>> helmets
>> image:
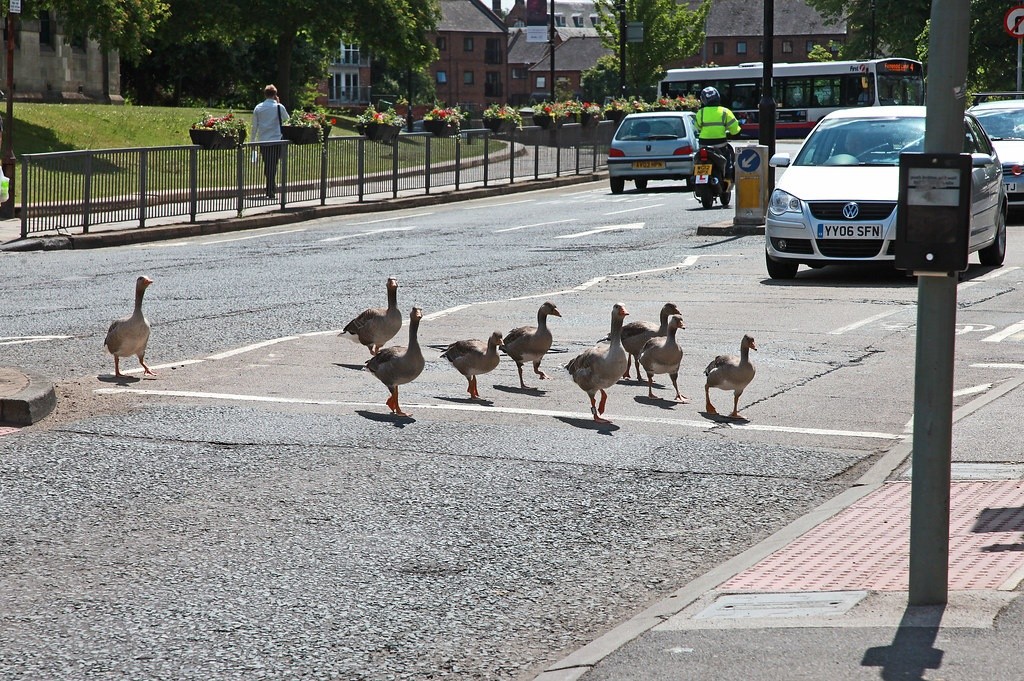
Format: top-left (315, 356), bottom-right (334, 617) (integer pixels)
top-left (700, 86), bottom-right (720, 106)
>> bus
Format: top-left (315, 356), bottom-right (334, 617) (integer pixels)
top-left (656, 57), bottom-right (926, 130)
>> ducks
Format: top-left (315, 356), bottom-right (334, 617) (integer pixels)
top-left (102, 275), bottom-right (161, 380)
top-left (637, 315), bottom-right (689, 403)
top-left (563, 302), bottom-right (630, 425)
top-left (702, 334), bottom-right (758, 420)
top-left (440, 329), bottom-right (505, 400)
top-left (498, 300), bottom-right (563, 390)
top-left (596, 302), bottom-right (683, 382)
top-left (364, 304), bottom-right (427, 418)
top-left (336, 276), bottom-right (403, 358)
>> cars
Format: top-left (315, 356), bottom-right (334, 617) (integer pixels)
top-left (965, 93), bottom-right (1024, 206)
top-left (765, 106), bottom-right (1007, 279)
top-left (607, 109), bottom-right (701, 193)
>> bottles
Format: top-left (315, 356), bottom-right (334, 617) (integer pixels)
top-left (250, 149), bottom-right (260, 162)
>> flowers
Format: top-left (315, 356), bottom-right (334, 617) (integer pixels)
top-left (483, 103), bottom-right (523, 131)
top-left (356, 104), bottom-right (406, 127)
top-left (531, 93), bottom-right (702, 122)
top-left (283, 108), bottom-right (336, 152)
top-left (423, 105), bottom-right (470, 140)
top-left (193, 106), bottom-right (246, 148)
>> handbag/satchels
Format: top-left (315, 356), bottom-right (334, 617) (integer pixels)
top-left (0, 166), bottom-right (10, 202)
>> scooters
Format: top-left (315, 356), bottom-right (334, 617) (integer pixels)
top-left (691, 119), bottom-right (747, 208)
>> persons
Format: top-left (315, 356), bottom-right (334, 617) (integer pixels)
top-left (250, 83), bottom-right (290, 201)
top-left (693, 86), bottom-right (742, 183)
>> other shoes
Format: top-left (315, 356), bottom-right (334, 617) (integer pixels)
top-left (266, 189), bottom-right (276, 198)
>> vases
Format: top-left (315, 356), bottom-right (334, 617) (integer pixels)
top-left (533, 106), bottom-right (698, 127)
top-left (354, 123), bottom-right (401, 141)
top-left (424, 121), bottom-right (464, 137)
top-left (189, 129), bottom-right (246, 148)
top-left (281, 126), bottom-right (332, 144)
top-left (482, 117), bottom-right (516, 133)
